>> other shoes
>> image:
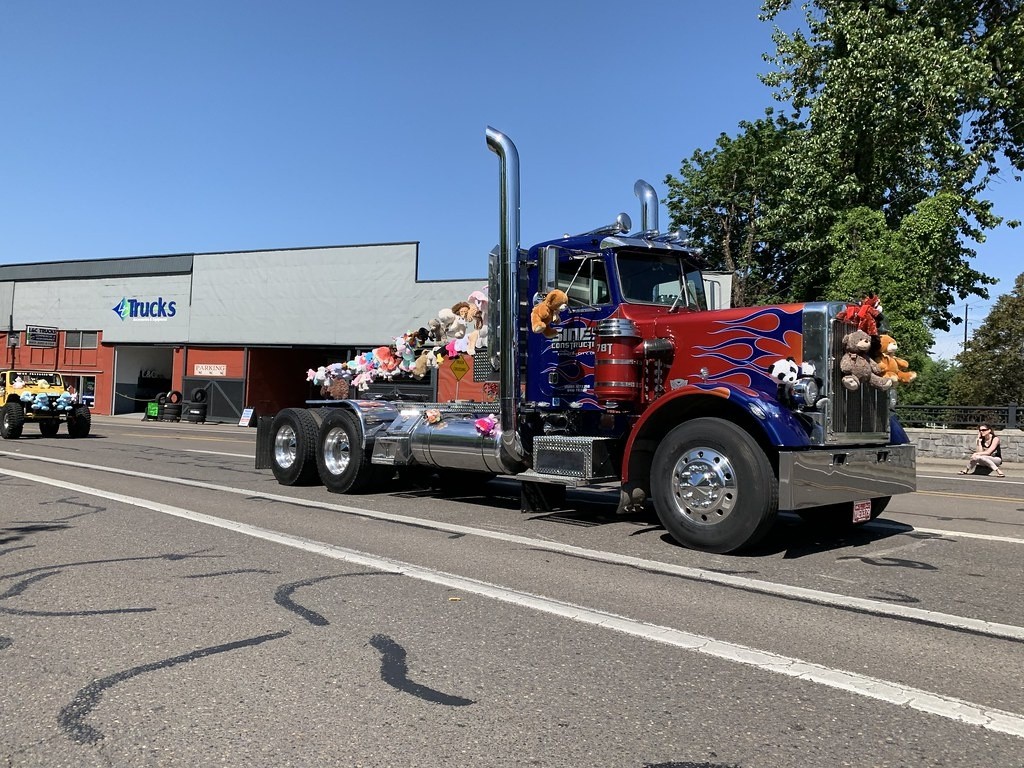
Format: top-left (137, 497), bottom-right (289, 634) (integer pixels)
top-left (957, 470), bottom-right (967, 475)
top-left (997, 473), bottom-right (1005, 477)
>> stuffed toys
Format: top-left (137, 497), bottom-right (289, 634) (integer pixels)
top-left (0, 386), bottom-right (6, 397)
top-left (306, 285), bottom-right (491, 401)
top-left (33, 379), bottom-right (50, 389)
top-left (474, 413), bottom-right (498, 436)
top-left (31, 392), bottom-right (50, 410)
top-left (858, 291), bottom-right (883, 336)
top-left (835, 304), bottom-right (859, 327)
top-left (878, 334), bottom-right (917, 387)
top-left (71, 390), bottom-right (78, 404)
top-left (56, 391), bottom-right (73, 411)
top-left (768, 356), bottom-right (823, 399)
top-left (13, 376), bottom-right (25, 389)
top-left (530, 289), bottom-right (568, 339)
top-left (425, 408), bottom-right (440, 423)
top-left (839, 329), bottom-right (893, 391)
top-left (20, 391), bottom-right (35, 401)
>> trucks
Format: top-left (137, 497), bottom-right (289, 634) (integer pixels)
top-left (255, 126), bottom-right (918, 555)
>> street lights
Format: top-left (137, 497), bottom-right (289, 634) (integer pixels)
top-left (10, 334), bottom-right (19, 369)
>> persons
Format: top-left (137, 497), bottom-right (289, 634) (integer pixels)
top-left (67, 384), bottom-right (76, 394)
top-left (957, 424), bottom-right (1006, 478)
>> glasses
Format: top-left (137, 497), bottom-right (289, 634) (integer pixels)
top-left (979, 428), bottom-right (990, 431)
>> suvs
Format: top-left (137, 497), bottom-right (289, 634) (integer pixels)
top-left (0, 369), bottom-right (91, 440)
